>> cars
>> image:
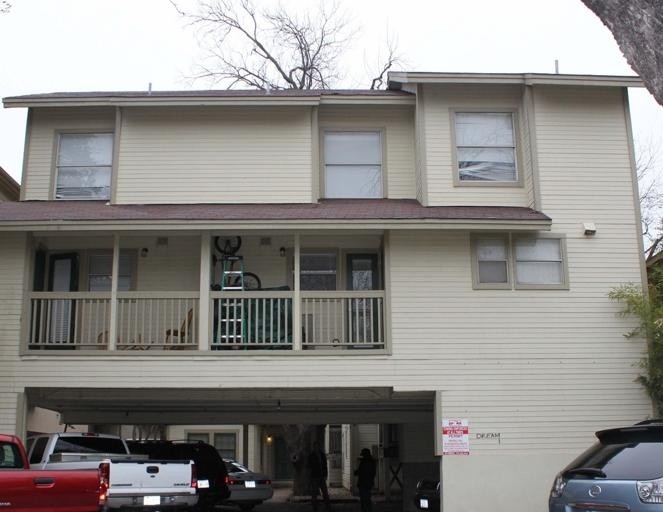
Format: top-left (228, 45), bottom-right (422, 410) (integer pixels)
top-left (219, 456), bottom-right (273, 512)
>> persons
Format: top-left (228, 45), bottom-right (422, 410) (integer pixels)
top-left (354, 448), bottom-right (376, 512)
top-left (309, 441), bottom-right (330, 512)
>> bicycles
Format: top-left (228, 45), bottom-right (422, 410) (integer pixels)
top-left (212, 235), bottom-right (261, 291)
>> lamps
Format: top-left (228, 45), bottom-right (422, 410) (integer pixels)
top-left (280, 247), bottom-right (285, 257)
top-left (584, 223), bottom-right (596, 236)
top-left (141, 248), bottom-right (148, 257)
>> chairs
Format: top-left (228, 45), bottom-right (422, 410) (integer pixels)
top-left (163, 307), bottom-right (193, 349)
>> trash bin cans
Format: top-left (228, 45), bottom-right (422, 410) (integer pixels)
top-left (414, 479), bottom-right (440, 512)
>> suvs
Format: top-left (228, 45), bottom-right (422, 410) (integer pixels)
top-left (547, 417), bottom-right (662, 512)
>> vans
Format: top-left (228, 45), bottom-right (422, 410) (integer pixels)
top-left (125, 439), bottom-right (230, 512)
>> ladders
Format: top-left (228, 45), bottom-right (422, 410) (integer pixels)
top-left (215, 256), bottom-right (247, 350)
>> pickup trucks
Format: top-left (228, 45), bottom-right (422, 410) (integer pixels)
top-left (25, 433), bottom-right (199, 512)
top-left (0, 432), bottom-right (109, 512)
